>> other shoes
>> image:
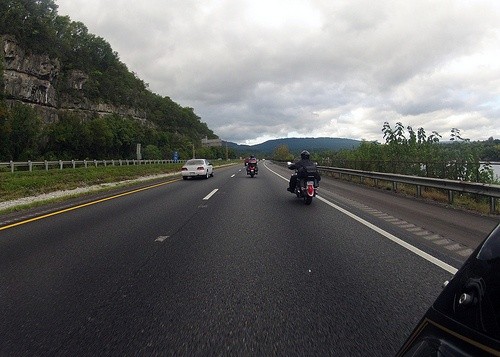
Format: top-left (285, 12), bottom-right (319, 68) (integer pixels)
top-left (287, 188), bottom-right (294, 192)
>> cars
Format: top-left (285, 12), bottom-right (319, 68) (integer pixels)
top-left (244, 157), bottom-right (250, 166)
top-left (181, 159), bottom-right (214, 180)
top-left (262, 159), bottom-right (265, 161)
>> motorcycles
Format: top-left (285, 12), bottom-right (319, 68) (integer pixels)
top-left (287, 162), bottom-right (320, 205)
top-left (246, 159), bottom-right (258, 178)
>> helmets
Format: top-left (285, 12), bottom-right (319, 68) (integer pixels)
top-left (301, 150), bottom-right (310, 155)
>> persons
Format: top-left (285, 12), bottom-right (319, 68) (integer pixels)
top-left (286, 149), bottom-right (318, 195)
top-left (246, 154), bottom-right (258, 175)
